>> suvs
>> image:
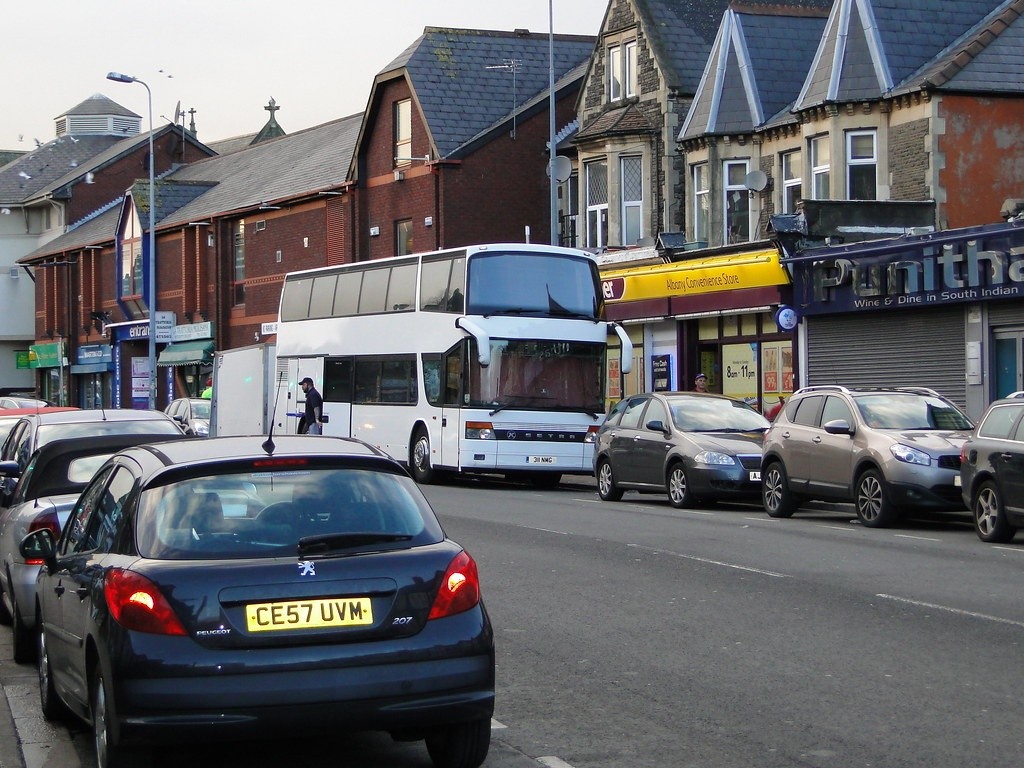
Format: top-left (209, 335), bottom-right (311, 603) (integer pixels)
top-left (760, 385), bottom-right (976, 527)
top-left (960, 384), bottom-right (1024, 545)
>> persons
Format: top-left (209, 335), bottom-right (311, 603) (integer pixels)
top-left (690, 373), bottom-right (710, 394)
top-left (297, 376), bottom-right (324, 435)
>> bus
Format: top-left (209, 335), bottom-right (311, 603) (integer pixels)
top-left (269, 243), bottom-right (632, 486)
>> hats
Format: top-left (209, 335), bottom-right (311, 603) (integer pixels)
top-left (696, 373), bottom-right (708, 379)
top-left (298, 378), bottom-right (312, 385)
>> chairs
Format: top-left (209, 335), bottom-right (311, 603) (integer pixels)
top-left (291, 480), bottom-right (354, 532)
top-left (173, 491), bottom-right (225, 535)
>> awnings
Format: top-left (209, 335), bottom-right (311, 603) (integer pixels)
top-left (157, 341), bottom-right (215, 368)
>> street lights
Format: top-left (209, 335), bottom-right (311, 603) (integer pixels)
top-left (107, 71), bottom-right (156, 411)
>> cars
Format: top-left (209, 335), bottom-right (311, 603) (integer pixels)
top-left (164, 398), bottom-right (211, 437)
top-left (0, 398), bottom-right (188, 669)
top-left (19, 435), bottom-right (496, 768)
top-left (593, 391), bottom-right (771, 509)
